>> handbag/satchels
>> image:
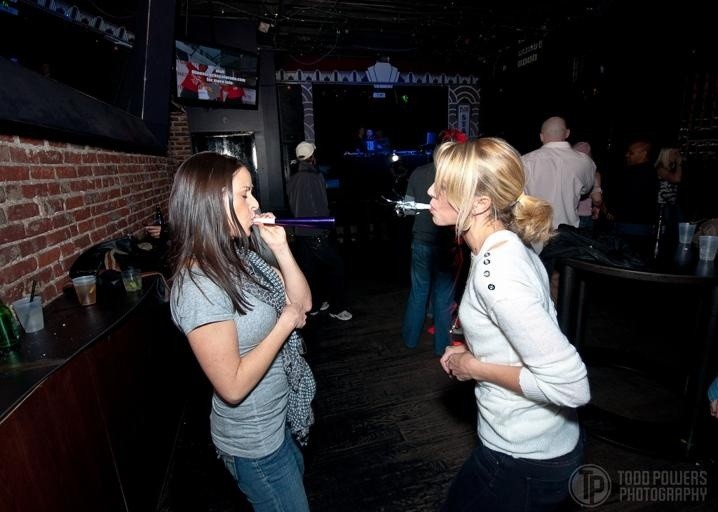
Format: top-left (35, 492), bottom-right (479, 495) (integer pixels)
top-left (539, 221), bottom-right (655, 270)
top-left (68, 235), bottom-right (173, 293)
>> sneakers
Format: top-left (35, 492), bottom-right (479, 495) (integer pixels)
top-left (329, 310), bottom-right (354, 321)
top-left (309, 300), bottom-right (330, 317)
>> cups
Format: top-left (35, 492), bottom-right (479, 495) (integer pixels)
top-left (679, 222), bottom-right (696, 243)
top-left (676, 242), bottom-right (692, 263)
top-left (12, 295), bottom-right (44, 334)
top-left (121, 266), bottom-right (142, 292)
top-left (699, 235), bottom-right (718, 261)
top-left (696, 260), bottom-right (716, 277)
top-left (71, 275), bottom-right (97, 306)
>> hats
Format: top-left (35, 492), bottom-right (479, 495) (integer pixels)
top-left (295, 141), bottom-right (318, 162)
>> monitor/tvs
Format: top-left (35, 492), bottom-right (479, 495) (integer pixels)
top-left (173, 37), bottom-right (260, 110)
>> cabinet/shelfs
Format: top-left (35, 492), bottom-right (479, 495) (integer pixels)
top-left (0, 279), bottom-right (221, 512)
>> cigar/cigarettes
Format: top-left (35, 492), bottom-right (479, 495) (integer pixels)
top-left (414, 203), bottom-right (431, 210)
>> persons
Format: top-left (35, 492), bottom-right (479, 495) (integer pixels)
top-left (400, 116), bottom-right (718, 356)
top-left (426, 137), bottom-right (592, 512)
top-left (144, 160), bottom-right (182, 245)
top-left (166, 151), bottom-right (315, 512)
top-left (286, 141), bottom-right (354, 321)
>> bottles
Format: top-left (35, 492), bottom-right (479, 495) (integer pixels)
top-left (653, 204), bottom-right (666, 242)
top-left (155, 203), bottom-right (165, 225)
top-left (0, 298), bottom-right (21, 349)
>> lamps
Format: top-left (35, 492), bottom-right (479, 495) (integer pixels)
top-left (366, 57), bottom-right (401, 88)
